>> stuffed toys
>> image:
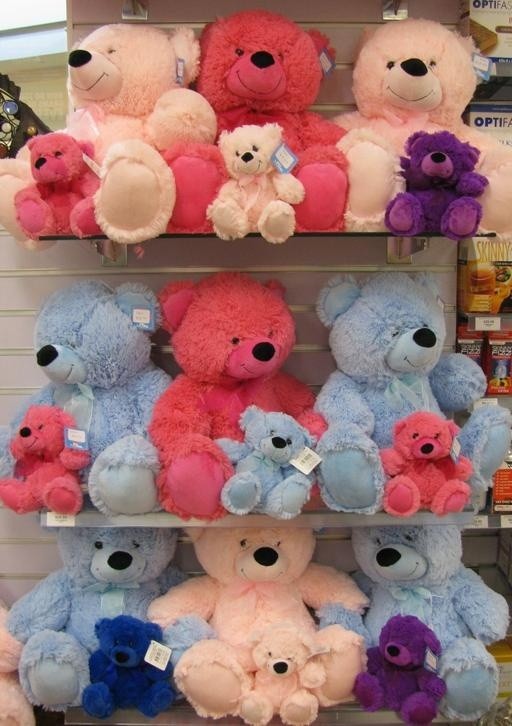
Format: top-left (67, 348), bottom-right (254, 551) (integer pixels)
top-left (212, 405), bottom-right (319, 522)
top-left (16, 132), bottom-right (103, 239)
top-left (149, 272), bottom-right (327, 522)
top-left (6, 528), bottom-right (216, 713)
top-left (239, 622), bottom-right (326, 726)
top-left (206, 122), bottom-right (305, 244)
top-left (385, 128), bottom-right (488, 240)
top-left (1, 404), bottom-right (90, 517)
top-left (161, 9), bottom-right (349, 236)
top-left (353, 614), bottom-right (446, 725)
top-left (1, 603), bottom-right (37, 726)
top-left (314, 268), bottom-right (511, 516)
top-left (378, 412), bottom-right (472, 516)
top-left (82, 615), bottom-right (176, 718)
top-left (318, 525), bottom-right (509, 722)
top-left (328, 16), bottom-right (511, 238)
top-left (1, 21), bottom-right (217, 252)
top-left (148, 526), bottom-right (370, 721)
top-left (1, 278), bottom-right (173, 518)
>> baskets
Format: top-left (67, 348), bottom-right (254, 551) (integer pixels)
top-left (494, 468), bottom-right (512, 502)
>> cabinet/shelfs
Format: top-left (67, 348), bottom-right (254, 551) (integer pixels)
top-left (3, 213), bottom-right (512, 726)
